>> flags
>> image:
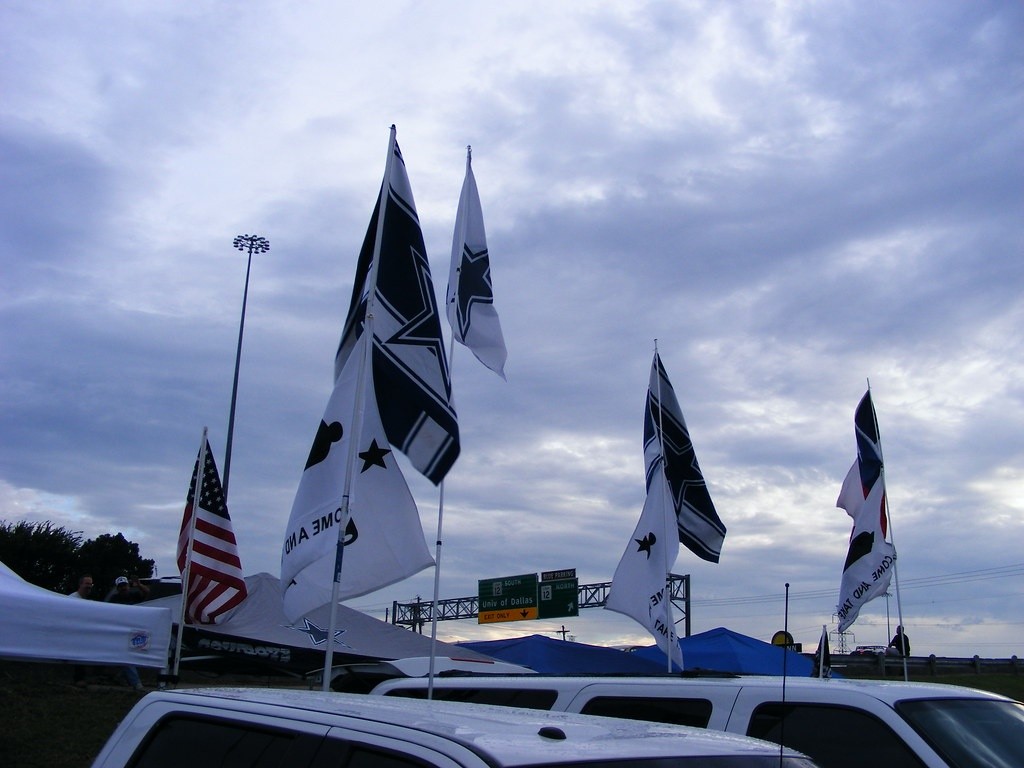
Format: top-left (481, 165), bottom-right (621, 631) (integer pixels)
top-left (444, 164), bottom-right (510, 381)
top-left (176, 437), bottom-right (248, 624)
top-left (280, 140), bottom-right (461, 624)
top-left (836, 389), bottom-right (894, 633)
top-left (603, 349), bottom-right (727, 670)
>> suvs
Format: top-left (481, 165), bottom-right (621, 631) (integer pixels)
top-left (323, 655), bottom-right (530, 708)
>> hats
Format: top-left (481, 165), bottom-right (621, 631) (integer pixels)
top-left (115, 576), bottom-right (128, 590)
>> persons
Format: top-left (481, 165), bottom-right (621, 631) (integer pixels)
top-left (68, 574), bottom-right (152, 700)
top-left (886, 624), bottom-right (911, 658)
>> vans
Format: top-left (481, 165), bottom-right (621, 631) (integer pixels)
top-left (88, 686), bottom-right (812, 768)
top-left (372, 672), bottom-right (1024, 768)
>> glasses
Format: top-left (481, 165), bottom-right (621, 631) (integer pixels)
top-left (82, 583), bottom-right (94, 586)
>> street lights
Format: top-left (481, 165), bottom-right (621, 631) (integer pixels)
top-left (222, 233), bottom-right (271, 502)
